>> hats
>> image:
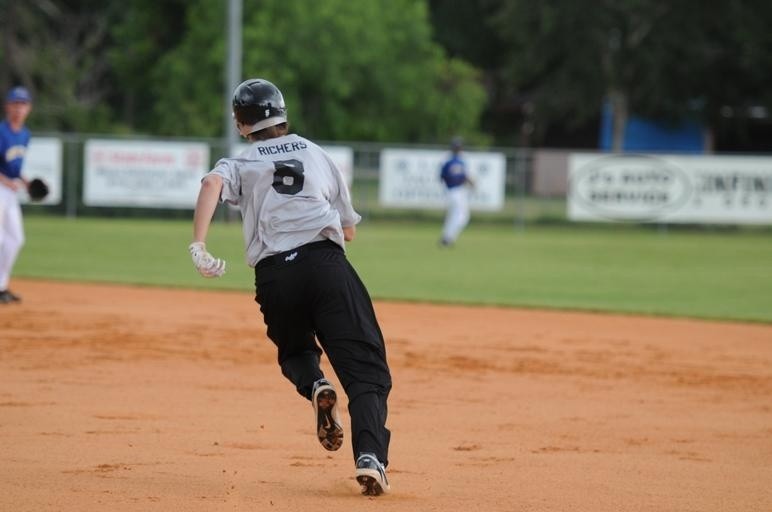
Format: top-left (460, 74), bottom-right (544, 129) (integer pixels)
top-left (8, 87), bottom-right (29, 103)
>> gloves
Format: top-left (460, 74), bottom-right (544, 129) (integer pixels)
top-left (188, 242), bottom-right (226, 277)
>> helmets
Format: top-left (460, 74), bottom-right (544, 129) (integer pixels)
top-left (232, 79), bottom-right (287, 136)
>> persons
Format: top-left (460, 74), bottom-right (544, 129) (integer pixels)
top-left (189, 78), bottom-right (393, 496)
top-left (0, 86), bottom-right (35, 302)
top-left (439, 138), bottom-right (476, 245)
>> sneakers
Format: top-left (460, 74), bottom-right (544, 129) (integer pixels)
top-left (354, 454), bottom-right (389, 495)
top-left (311, 378), bottom-right (344, 451)
top-left (0, 292), bottom-right (21, 301)
